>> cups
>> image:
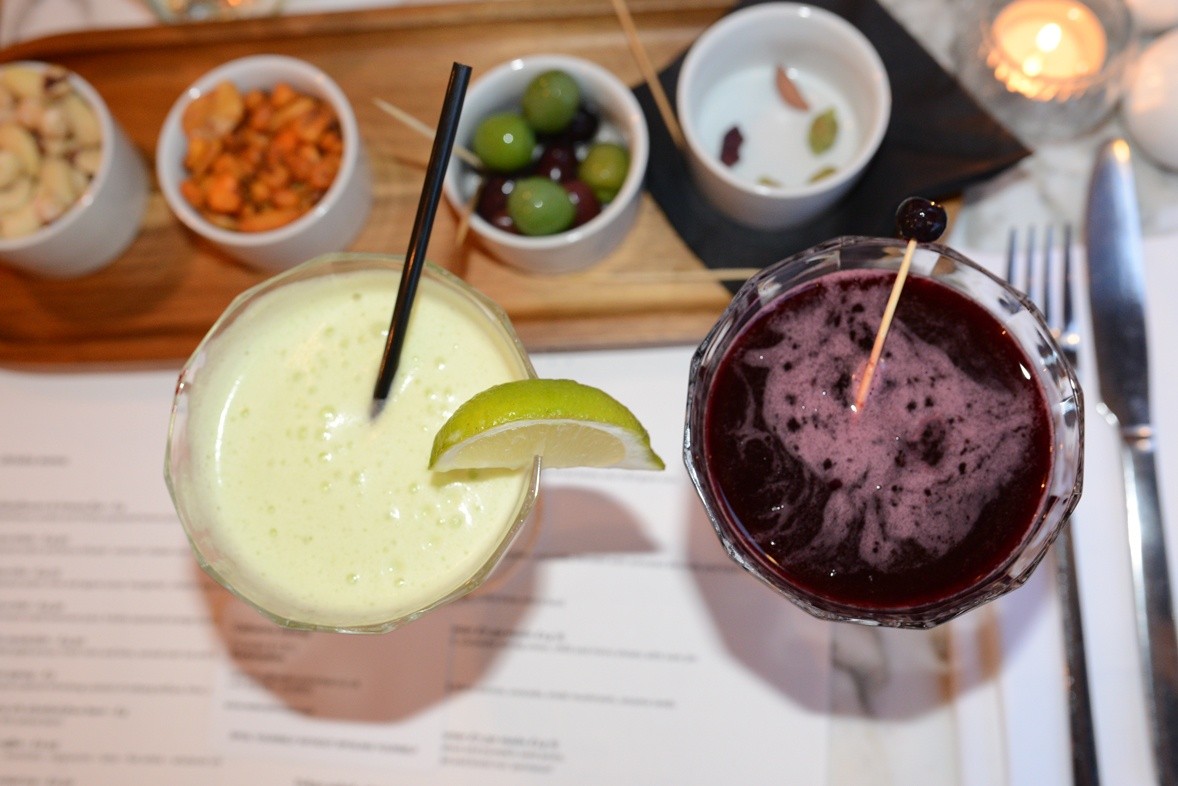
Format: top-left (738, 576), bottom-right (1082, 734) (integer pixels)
top-left (163, 251), bottom-right (538, 636)
top-left (684, 233), bottom-right (1084, 631)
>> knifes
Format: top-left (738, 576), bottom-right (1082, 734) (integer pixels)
top-left (1086, 135), bottom-right (1178, 786)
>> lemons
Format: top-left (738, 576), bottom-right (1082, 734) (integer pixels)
top-left (423, 378), bottom-right (667, 471)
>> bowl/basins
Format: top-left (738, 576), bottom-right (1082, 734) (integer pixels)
top-left (676, 0), bottom-right (891, 234)
top-left (1, 60), bottom-right (146, 276)
top-left (442, 57), bottom-right (650, 276)
top-left (155, 54), bottom-right (369, 273)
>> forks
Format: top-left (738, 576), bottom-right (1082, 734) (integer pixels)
top-left (1004, 222), bottom-right (1102, 785)
